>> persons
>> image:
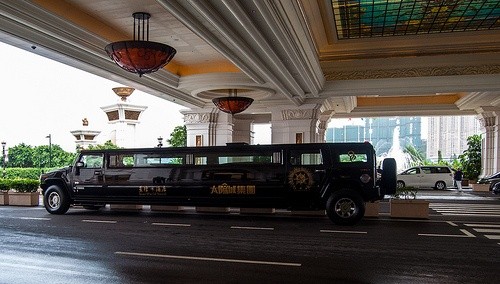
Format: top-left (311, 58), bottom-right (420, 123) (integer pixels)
top-left (454, 168), bottom-right (464, 192)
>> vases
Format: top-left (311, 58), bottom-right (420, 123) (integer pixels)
top-left (378, 197), bottom-right (390, 213)
top-left (364, 201), bottom-right (378, 216)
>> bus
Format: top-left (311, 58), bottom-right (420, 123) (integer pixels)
top-left (38, 140), bottom-right (397, 228)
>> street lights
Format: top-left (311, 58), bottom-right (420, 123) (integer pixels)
top-left (45, 133), bottom-right (51, 167)
top-left (0, 141), bottom-right (7, 179)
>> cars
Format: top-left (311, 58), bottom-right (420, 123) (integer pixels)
top-left (475, 171), bottom-right (500, 196)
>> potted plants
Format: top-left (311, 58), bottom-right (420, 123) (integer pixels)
top-left (0, 176), bottom-right (10, 206)
top-left (471, 178), bottom-right (492, 191)
top-left (7, 176), bottom-right (40, 206)
top-left (390, 184), bottom-right (429, 219)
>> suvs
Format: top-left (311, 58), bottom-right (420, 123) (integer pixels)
top-left (395, 165), bottom-right (455, 191)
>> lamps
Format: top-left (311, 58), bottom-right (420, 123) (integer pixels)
top-left (210, 88), bottom-right (254, 115)
top-left (104, 12), bottom-right (176, 78)
